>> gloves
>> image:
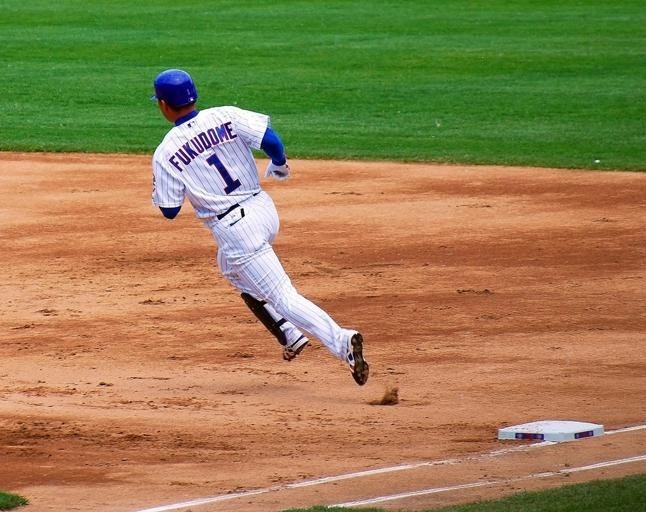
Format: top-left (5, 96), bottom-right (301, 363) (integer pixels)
top-left (264, 162), bottom-right (290, 180)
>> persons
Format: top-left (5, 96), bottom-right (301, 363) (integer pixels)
top-left (149, 69), bottom-right (370, 386)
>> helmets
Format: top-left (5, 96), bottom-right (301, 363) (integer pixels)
top-left (151, 69), bottom-right (197, 106)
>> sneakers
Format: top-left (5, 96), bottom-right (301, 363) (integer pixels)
top-left (345, 331), bottom-right (369, 385)
top-left (283, 335), bottom-right (308, 360)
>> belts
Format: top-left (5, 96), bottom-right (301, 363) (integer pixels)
top-left (217, 203), bottom-right (240, 219)
